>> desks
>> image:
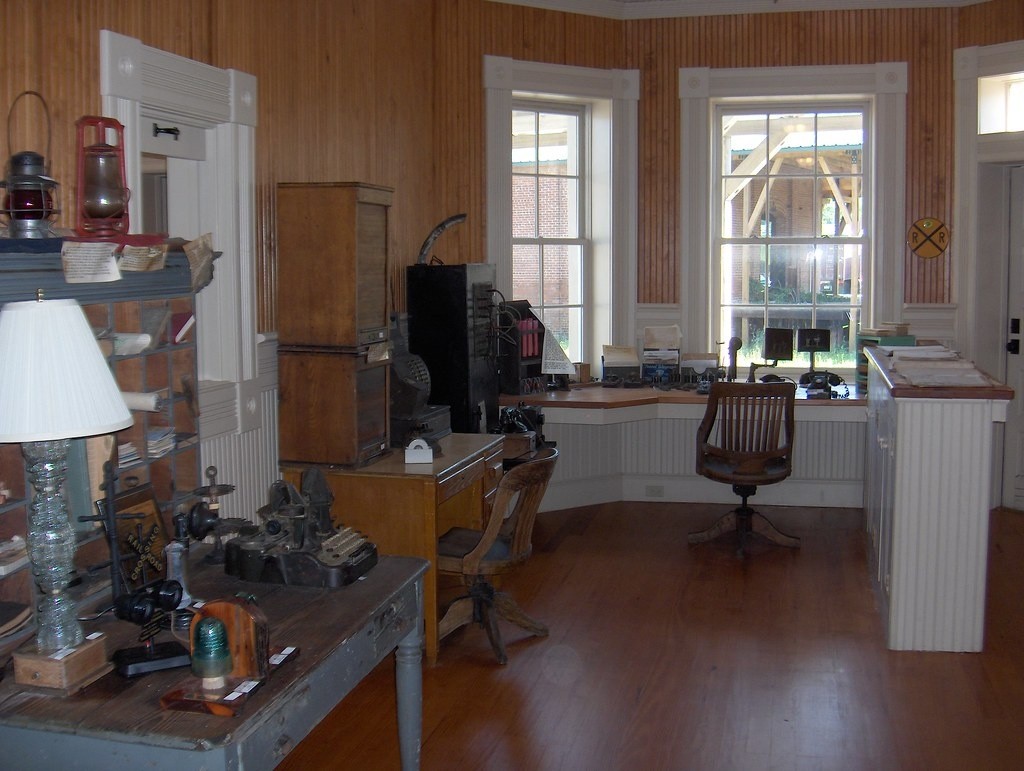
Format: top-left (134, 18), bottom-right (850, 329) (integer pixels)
top-left (500, 383), bottom-right (870, 517)
top-left (282, 432), bottom-right (507, 665)
top-left (1, 548), bottom-right (430, 771)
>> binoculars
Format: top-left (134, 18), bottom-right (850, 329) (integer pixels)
top-left (111, 578), bottom-right (184, 627)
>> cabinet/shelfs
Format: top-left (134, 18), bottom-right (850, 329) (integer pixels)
top-left (862, 346), bottom-right (1014, 653)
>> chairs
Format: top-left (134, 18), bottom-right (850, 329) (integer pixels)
top-left (393, 447), bottom-right (560, 666)
top-left (686, 382), bottom-right (803, 551)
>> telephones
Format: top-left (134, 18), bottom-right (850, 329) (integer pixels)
top-left (799, 370), bottom-right (841, 400)
top-left (498, 406), bottom-right (528, 434)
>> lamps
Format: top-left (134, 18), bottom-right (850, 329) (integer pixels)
top-left (0, 288), bottom-right (134, 698)
top-left (2, 92), bottom-right (65, 243)
top-left (74, 116), bottom-right (131, 241)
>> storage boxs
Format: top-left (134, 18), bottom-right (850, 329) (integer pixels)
top-left (275, 179), bottom-right (395, 469)
top-left (391, 406), bottom-right (454, 449)
top-left (505, 431), bottom-right (538, 461)
